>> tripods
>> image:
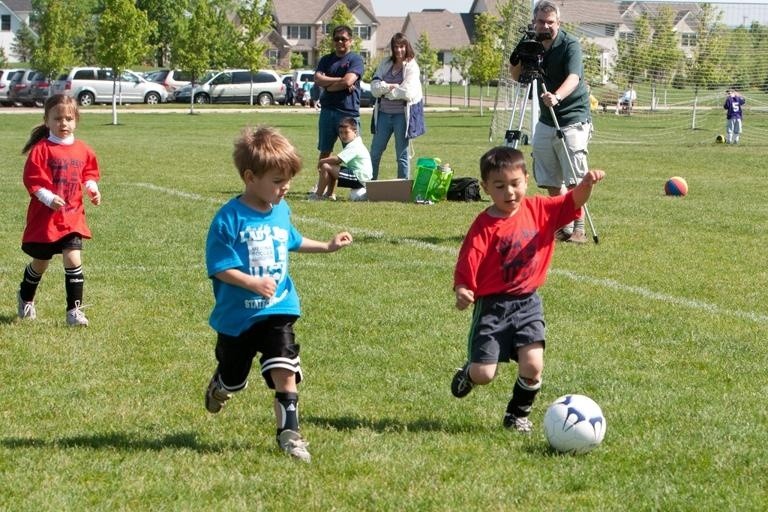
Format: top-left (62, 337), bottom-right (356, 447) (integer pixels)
top-left (504, 71), bottom-right (600, 244)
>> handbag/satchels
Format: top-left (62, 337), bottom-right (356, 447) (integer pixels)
top-left (408, 162), bottom-right (454, 205)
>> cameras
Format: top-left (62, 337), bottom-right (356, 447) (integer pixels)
top-left (516, 24), bottom-right (552, 70)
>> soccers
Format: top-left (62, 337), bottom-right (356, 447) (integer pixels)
top-left (664, 176), bottom-right (689, 198)
top-left (715, 135), bottom-right (727, 145)
top-left (348, 188), bottom-right (367, 202)
top-left (542, 392), bottom-right (607, 456)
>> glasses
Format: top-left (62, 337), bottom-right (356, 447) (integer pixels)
top-left (332, 36), bottom-right (350, 42)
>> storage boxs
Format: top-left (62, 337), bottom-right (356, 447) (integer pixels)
top-left (366, 180), bottom-right (415, 202)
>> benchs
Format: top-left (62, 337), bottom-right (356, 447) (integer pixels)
top-left (598, 92), bottom-right (634, 115)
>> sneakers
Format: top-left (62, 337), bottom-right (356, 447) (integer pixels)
top-left (329, 193), bottom-right (337, 200)
top-left (307, 183), bottom-right (328, 195)
top-left (304, 193), bottom-right (325, 200)
top-left (205, 371), bottom-right (232, 413)
top-left (16, 289), bottom-right (37, 321)
top-left (65, 299), bottom-right (94, 326)
top-left (279, 429), bottom-right (312, 464)
top-left (553, 230), bottom-right (571, 241)
top-left (451, 360), bottom-right (475, 398)
top-left (566, 230), bottom-right (589, 243)
top-left (503, 412), bottom-right (537, 439)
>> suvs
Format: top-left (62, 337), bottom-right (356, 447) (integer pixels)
top-left (9, 70), bottom-right (37, 106)
top-left (294, 70), bottom-right (376, 107)
top-left (143, 70), bottom-right (170, 85)
top-left (0, 68), bottom-right (24, 106)
top-left (173, 68), bottom-right (286, 106)
top-left (52, 67), bottom-right (169, 106)
top-left (31, 71), bottom-right (58, 108)
top-left (163, 69), bottom-right (218, 100)
top-left (282, 75), bottom-right (293, 85)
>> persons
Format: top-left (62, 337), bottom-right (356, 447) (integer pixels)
top-left (586, 85), bottom-right (599, 111)
top-left (302, 77), bottom-right (312, 108)
top-left (305, 117), bottom-right (373, 201)
top-left (510, 1), bottom-right (592, 245)
top-left (308, 25), bottom-right (364, 194)
top-left (724, 89), bottom-right (745, 143)
top-left (370, 33), bottom-right (426, 179)
top-left (203, 128), bottom-right (353, 466)
top-left (311, 84), bottom-right (321, 109)
top-left (16, 94), bottom-right (102, 328)
top-left (451, 148), bottom-right (605, 432)
top-left (616, 80), bottom-right (637, 117)
top-left (284, 78), bottom-right (295, 106)
